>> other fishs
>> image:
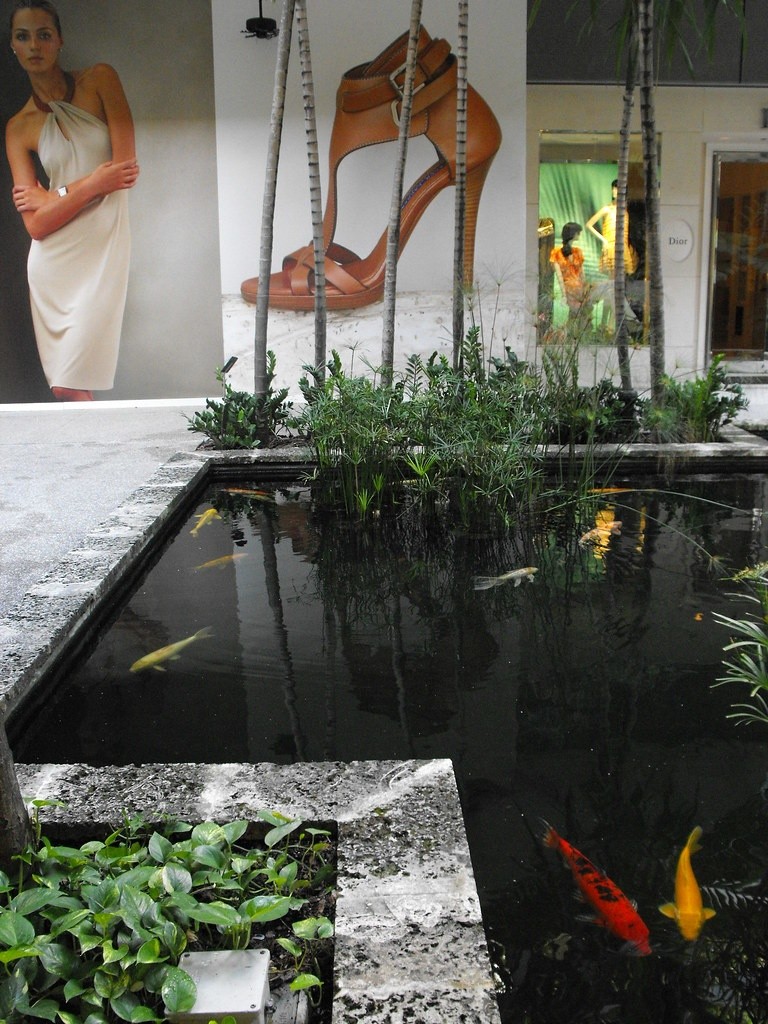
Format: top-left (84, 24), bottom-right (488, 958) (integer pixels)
top-left (129, 625), bottom-right (216, 673)
top-left (578, 521), bottom-right (623, 551)
top-left (534, 814), bottom-right (652, 958)
top-left (187, 552), bottom-right (248, 578)
top-left (189, 508), bottom-right (222, 537)
top-left (657, 825), bottom-right (717, 942)
top-left (587, 486), bottom-right (656, 496)
top-left (218, 487), bottom-right (275, 502)
top-left (470, 566), bottom-right (539, 590)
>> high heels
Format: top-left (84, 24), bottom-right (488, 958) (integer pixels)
top-left (240, 24), bottom-right (503, 312)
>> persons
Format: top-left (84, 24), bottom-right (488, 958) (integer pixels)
top-left (550, 222), bottom-right (594, 330)
top-left (586, 179), bottom-right (633, 284)
top-left (3, 0), bottom-right (139, 404)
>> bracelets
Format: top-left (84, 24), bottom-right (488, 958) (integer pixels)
top-left (57, 186), bottom-right (67, 197)
